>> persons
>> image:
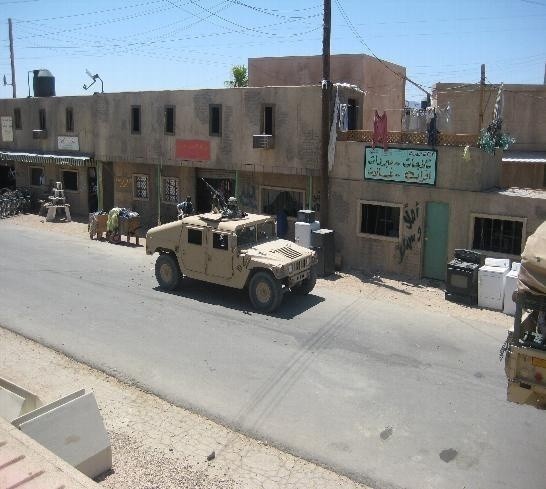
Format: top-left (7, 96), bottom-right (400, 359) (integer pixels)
top-left (218, 196), bottom-right (241, 219)
top-left (176, 196), bottom-right (194, 218)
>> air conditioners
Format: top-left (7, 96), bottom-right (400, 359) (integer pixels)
top-left (254, 135), bottom-right (273, 149)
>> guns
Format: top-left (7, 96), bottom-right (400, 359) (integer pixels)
top-left (201, 177), bottom-right (227, 214)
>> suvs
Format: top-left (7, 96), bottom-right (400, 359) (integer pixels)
top-left (145, 177), bottom-right (318, 316)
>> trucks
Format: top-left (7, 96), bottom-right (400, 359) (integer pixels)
top-left (497, 219), bottom-right (544, 410)
